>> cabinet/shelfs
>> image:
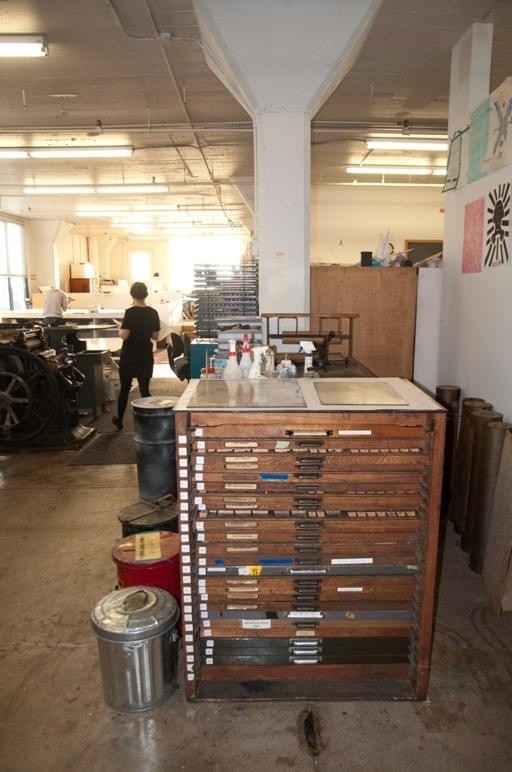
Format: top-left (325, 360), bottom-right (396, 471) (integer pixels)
top-left (172, 378), bottom-right (448, 701)
top-left (2, 309), bottom-right (125, 338)
top-left (261, 313), bottom-right (359, 366)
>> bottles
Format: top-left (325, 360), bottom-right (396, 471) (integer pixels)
top-left (223, 351), bottom-right (243, 380)
top-left (239, 349), bottom-right (253, 377)
top-left (276, 361), bottom-right (296, 378)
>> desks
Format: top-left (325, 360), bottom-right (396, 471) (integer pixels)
top-left (79, 338), bottom-right (125, 401)
top-left (190, 337), bottom-right (218, 379)
top-left (297, 357), bottom-right (377, 377)
top-left (65, 324), bottom-right (116, 338)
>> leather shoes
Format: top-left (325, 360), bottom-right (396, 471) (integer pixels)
top-left (113, 416), bottom-right (122, 429)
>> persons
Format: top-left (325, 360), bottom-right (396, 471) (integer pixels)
top-left (42, 289), bottom-right (68, 326)
top-left (151, 272), bottom-right (161, 292)
top-left (112, 282), bottom-right (160, 429)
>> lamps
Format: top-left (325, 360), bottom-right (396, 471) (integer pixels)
top-left (20, 168), bottom-right (169, 195)
top-left (363, 134), bottom-right (448, 151)
top-left (343, 149), bottom-right (447, 176)
top-left (0, 35), bottom-right (49, 57)
top-left (0, 145), bottom-right (134, 159)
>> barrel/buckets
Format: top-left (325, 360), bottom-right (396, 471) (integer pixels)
top-left (127, 395), bottom-right (176, 502)
top-left (111, 494), bottom-right (180, 594)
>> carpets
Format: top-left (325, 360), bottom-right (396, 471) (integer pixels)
top-left (84, 378), bottom-right (188, 432)
top-left (64, 433), bottom-right (137, 465)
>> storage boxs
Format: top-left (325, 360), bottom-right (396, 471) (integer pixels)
top-left (71, 278), bottom-right (95, 293)
top-left (70, 263), bottom-right (94, 278)
